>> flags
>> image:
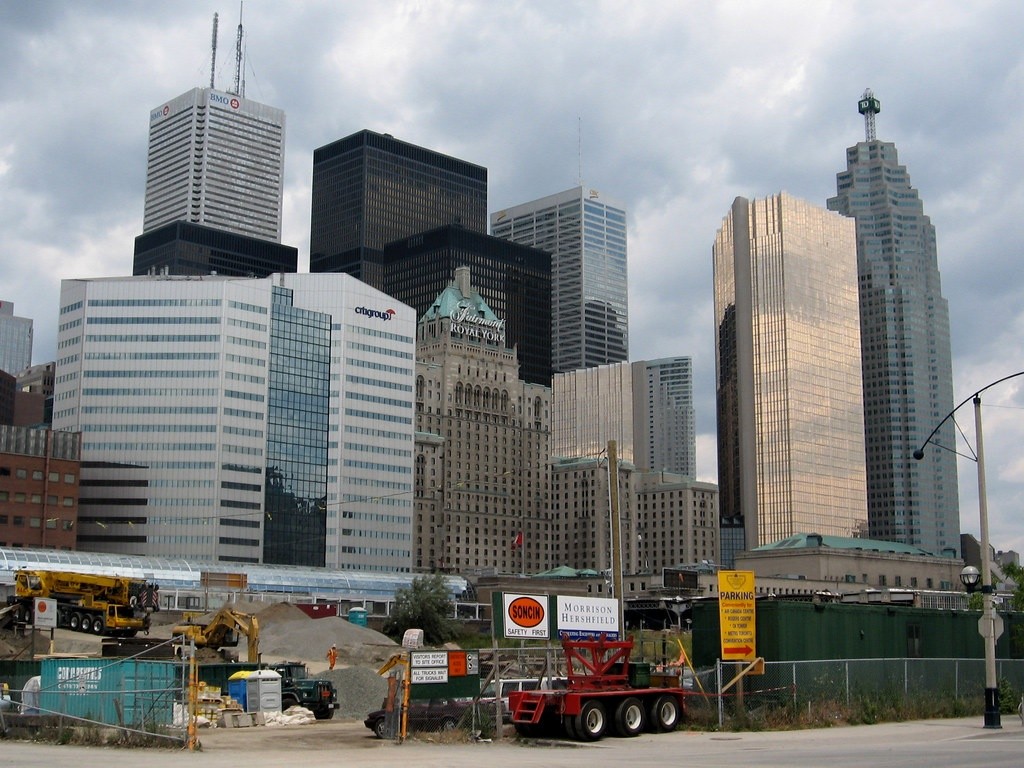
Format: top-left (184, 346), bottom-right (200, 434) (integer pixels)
top-left (511, 530), bottom-right (522, 546)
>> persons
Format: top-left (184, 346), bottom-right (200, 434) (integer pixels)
top-left (325, 643), bottom-right (339, 671)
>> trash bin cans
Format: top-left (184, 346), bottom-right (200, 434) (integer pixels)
top-left (228, 670), bottom-right (258, 712)
top-left (245, 670), bottom-right (282, 714)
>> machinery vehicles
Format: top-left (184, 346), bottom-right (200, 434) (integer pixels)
top-left (507, 633), bottom-right (684, 743)
top-left (171, 607), bottom-right (340, 720)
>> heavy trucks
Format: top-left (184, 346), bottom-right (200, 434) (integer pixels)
top-left (6, 569), bottom-right (159, 637)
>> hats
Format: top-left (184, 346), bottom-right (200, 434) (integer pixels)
top-left (332, 644), bottom-right (336, 647)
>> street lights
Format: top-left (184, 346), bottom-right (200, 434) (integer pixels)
top-left (959, 566), bottom-right (1003, 729)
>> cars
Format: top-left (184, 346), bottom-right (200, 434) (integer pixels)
top-left (364, 697), bottom-right (506, 739)
top-left (444, 678), bottom-right (568, 711)
